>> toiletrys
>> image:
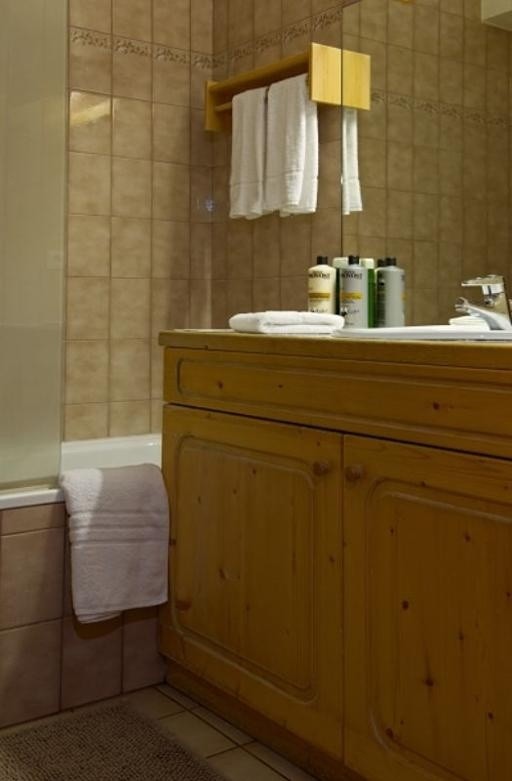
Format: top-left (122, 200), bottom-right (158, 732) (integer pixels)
top-left (306, 255), bottom-right (406, 328)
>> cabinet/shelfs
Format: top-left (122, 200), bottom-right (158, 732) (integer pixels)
top-left (160, 330), bottom-right (511, 781)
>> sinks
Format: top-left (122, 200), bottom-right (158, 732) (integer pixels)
top-left (332, 322), bottom-right (512, 341)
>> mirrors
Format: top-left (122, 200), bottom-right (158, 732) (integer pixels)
top-left (341, 0), bottom-right (512, 329)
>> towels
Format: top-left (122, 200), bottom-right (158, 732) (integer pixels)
top-left (228, 86), bottom-right (274, 220)
top-left (57, 464), bottom-right (169, 624)
top-left (262, 71), bottom-right (319, 218)
top-left (228, 311), bottom-right (344, 335)
top-left (449, 315), bottom-right (489, 329)
top-left (341, 107), bottom-right (363, 216)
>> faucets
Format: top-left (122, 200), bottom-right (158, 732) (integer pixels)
top-left (454, 274), bottom-right (511, 331)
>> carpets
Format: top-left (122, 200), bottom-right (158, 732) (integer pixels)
top-left (0, 700), bottom-right (228, 781)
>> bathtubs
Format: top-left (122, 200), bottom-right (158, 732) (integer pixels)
top-left (0, 432), bottom-right (162, 509)
